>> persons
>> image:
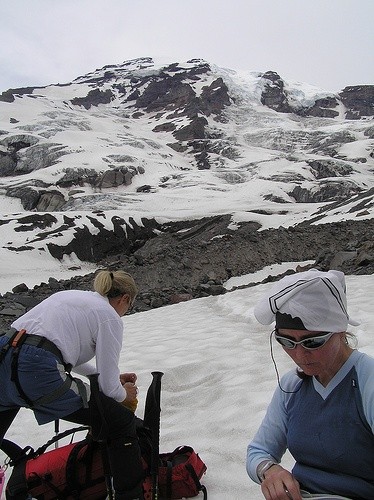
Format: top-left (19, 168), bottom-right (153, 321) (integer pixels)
top-left (0, 271), bottom-right (142, 499)
top-left (245, 268), bottom-right (374, 499)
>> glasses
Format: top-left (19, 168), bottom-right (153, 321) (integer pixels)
top-left (274, 331), bottom-right (334, 350)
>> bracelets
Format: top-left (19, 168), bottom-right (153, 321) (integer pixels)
top-left (257, 461), bottom-right (278, 479)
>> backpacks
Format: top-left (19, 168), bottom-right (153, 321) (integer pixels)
top-left (0, 416), bottom-right (208, 500)
